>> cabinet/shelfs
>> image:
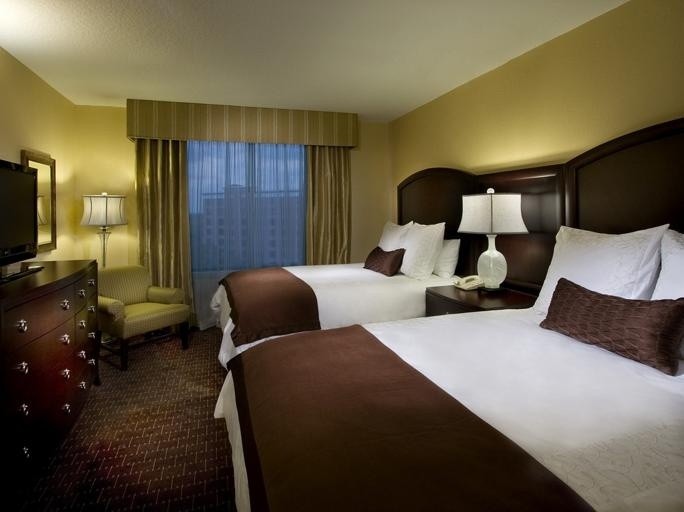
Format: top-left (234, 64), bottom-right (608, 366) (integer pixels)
top-left (0, 259), bottom-right (100, 511)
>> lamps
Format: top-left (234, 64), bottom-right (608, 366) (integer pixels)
top-left (77, 192), bottom-right (129, 267)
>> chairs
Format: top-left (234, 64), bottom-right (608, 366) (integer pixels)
top-left (98, 264), bottom-right (193, 371)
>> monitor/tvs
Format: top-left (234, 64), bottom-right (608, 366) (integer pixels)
top-left (0, 159), bottom-right (44, 285)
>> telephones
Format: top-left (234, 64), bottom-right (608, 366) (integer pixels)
top-left (453, 275), bottom-right (481, 291)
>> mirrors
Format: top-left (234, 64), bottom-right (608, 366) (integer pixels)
top-left (18, 145), bottom-right (57, 255)
